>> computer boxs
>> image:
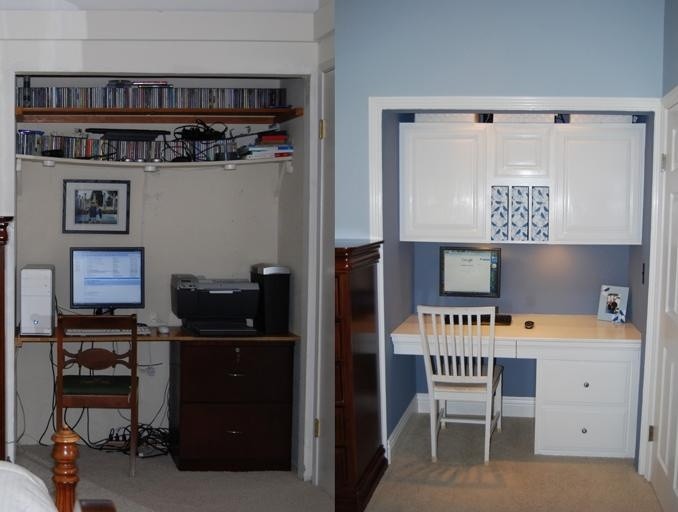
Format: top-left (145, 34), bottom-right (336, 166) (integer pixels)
top-left (19, 263), bottom-right (53, 338)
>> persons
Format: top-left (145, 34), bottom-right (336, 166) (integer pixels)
top-left (76, 190), bottom-right (118, 224)
top-left (610, 298), bottom-right (617, 313)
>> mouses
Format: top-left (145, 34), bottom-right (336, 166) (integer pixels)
top-left (525, 319), bottom-right (537, 331)
top-left (156, 324), bottom-right (170, 334)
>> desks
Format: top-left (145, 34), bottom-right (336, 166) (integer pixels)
top-left (16, 326), bottom-right (300, 472)
top-left (390, 313), bottom-right (642, 459)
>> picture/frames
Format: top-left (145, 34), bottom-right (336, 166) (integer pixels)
top-left (597, 285), bottom-right (630, 323)
top-left (62, 179), bottom-right (130, 234)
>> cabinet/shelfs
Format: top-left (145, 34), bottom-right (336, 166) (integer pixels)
top-left (334, 238), bottom-right (389, 512)
top-left (15, 74), bottom-right (304, 172)
top-left (398, 123), bottom-right (646, 247)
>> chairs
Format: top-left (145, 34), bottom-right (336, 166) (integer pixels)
top-left (417, 305), bottom-right (504, 463)
top-left (57, 313), bottom-right (139, 478)
top-left (0, 426), bottom-right (117, 512)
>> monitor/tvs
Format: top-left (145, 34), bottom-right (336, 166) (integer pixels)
top-left (70, 246), bottom-right (145, 314)
top-left (439, 245), bottom-right (502, 298)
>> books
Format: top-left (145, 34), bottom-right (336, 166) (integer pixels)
top-left (17, 133), bottom-right (293, 160)
top-left (16, 78), bottom-right (288, 108)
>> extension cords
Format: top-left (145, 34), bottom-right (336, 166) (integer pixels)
top-left (106, 440), bottom-right (129, 448)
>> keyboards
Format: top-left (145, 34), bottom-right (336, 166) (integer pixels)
top-left (66, 327), bottom-right (153, 336)
top-left (445, 314), bottom-right (513, 326)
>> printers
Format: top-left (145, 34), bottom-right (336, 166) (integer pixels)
top-left (169, 272), bottom-right (261, 336)
